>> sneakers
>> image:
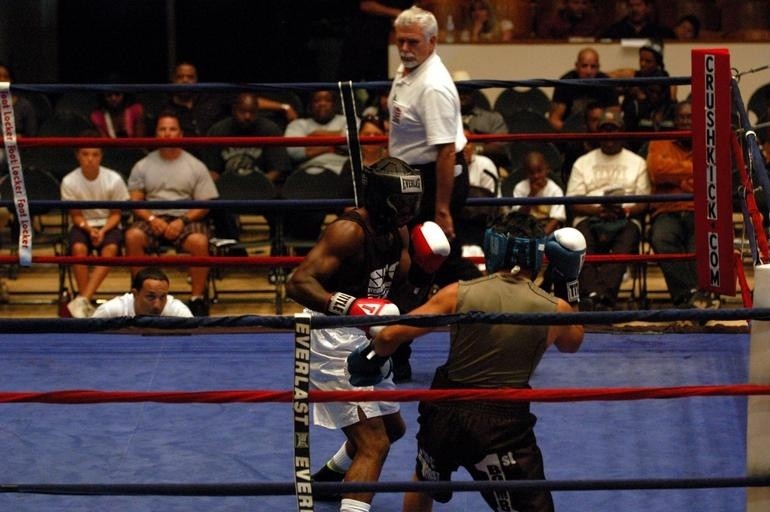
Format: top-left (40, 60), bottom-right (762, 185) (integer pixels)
top-left (391, 358), bottom-right (413, 382)
top-left (311, 475), bottom-right (342, 502)
top-left (64, 291), bottom-right (211, 320)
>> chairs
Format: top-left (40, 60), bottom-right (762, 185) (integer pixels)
top-left (0, 70), bottom-right (770, 311)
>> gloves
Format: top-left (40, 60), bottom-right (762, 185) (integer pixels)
top-left (324, 291), bottom-right (400, 339)
top-left (347, 336), bottom-right (391, 388)
top-left (542, 227), bottom-right (588, 307)
top-left (408, 221), bottom-right (452, 288)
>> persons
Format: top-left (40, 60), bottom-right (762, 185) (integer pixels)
top-left (7, 62), bottom-right (391, 183)
top-left (3, 43), bottom-right (770, 330)
top-left (60, 108), bottom-right (220, 318)
top-left (386, 7), bottom-right (485, 383)
top-left (453, 44), bottom-right (721, 308)
top-left (284, 155), bottom-right (453, 512)
top-left (344, 209), bottom-right (590, 510)
top-left (358, 1), bottom-right (770, 43)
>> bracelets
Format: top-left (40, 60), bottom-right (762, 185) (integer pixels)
top-left (146, 215), bottom-right (155, 224)
top-left (281, 104), bottom-right (291, 112)
top-left (181, 215), bottom-right (190, 226)
top-left (79, 222), bottom-right (86, 228)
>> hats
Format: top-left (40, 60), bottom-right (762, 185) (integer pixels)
top-left (596, 110), bottom-right (625, 132)
